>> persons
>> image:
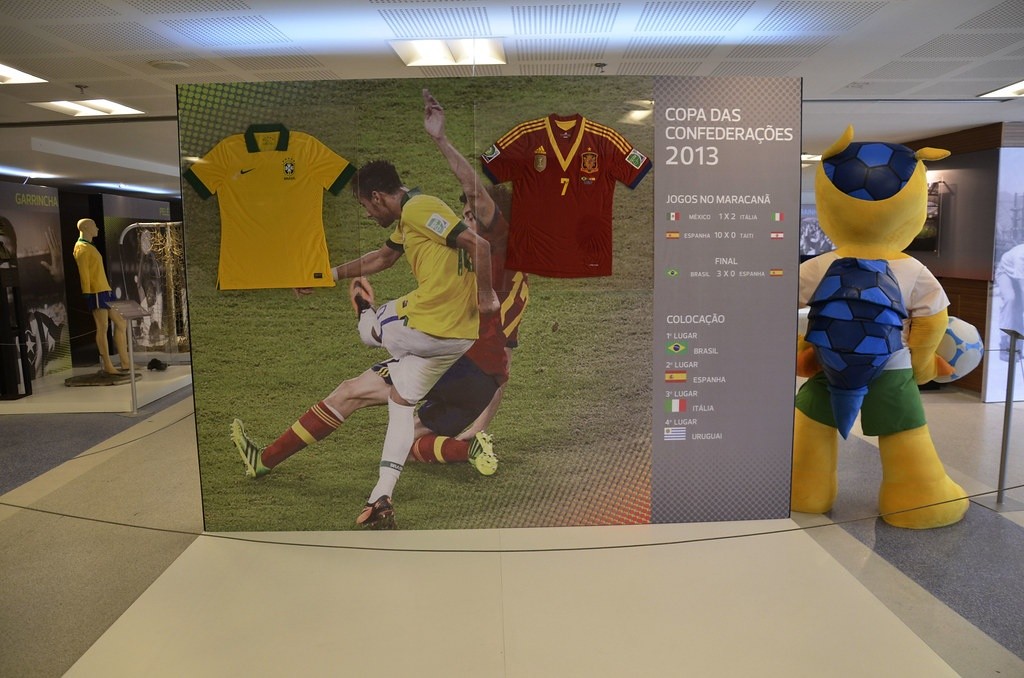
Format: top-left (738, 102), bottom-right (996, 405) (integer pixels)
top-left (230, 88), bottom-right (530, 480)
top-left (145, 282), bottom-right (163, 342)
top-left (292, 158), bottom-right (499, 530)
top-left (0, 216), bottom-right (65, 380)
top-left (73, 219), bottom-right (144, 374)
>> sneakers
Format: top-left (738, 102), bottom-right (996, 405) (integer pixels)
top-left (356, 494), bottom-right (394, 525)
top-left (349, 276), bottom-right (375, 318)
top-left (229, 417), bottom-right (273, 479)
top-left (468, 429), bottom-right (499, 476)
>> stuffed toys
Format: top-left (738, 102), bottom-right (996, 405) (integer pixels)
top-left (789, 126), bottom-right (969, 529)
